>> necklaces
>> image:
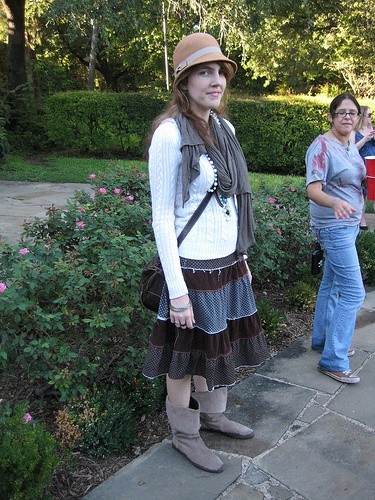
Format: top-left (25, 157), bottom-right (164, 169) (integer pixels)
top-left (333, 131), bottom-right (350, 151)
top-left (204, 110), bottom-right (232, 220)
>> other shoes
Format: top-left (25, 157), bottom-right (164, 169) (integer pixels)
top-left (348, 349), bottom-right (355, 356)
top-left (319, 366), bottom-right (361, 383)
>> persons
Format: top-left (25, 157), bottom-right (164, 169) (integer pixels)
top-left (306, 93), bottom-right (375, 383)
top-left (148, 32), bottom-right (271, 473)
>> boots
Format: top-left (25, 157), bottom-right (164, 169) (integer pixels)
top-left (166, 395), bottom-right (224, 473)
top-left (191, 386), bottom-right (254, 439)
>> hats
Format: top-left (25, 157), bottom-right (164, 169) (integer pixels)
top-left (172, 32), bottom-right (237, 91)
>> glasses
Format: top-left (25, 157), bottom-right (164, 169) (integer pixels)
top-left (366, 114), bottom-right (371, 119)
top-left (332, 110), bottom-right (360, 118)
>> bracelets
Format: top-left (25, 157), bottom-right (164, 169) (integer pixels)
top-left (169, 299), bottom-right (192, 312)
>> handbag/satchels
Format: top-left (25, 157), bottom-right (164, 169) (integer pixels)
top-left (139, 265), bottom-right (166, 313)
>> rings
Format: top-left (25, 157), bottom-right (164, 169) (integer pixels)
top-left (175, 321), bottom-right (186, 327)
